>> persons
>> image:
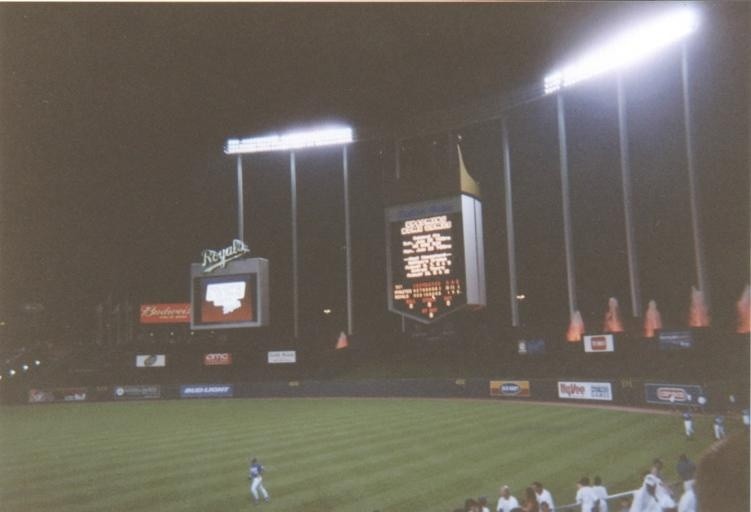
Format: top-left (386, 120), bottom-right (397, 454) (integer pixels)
top-left (246, 457), bottom-right (272, 506)
top-left (451, 450), bottom-right (700, 512)
top-left (681, 407), bottom-right (698, 441)
top-left (739, 404), bottom-right (751, 436)
top-left (712, 411), bottom-right (727, 443)
